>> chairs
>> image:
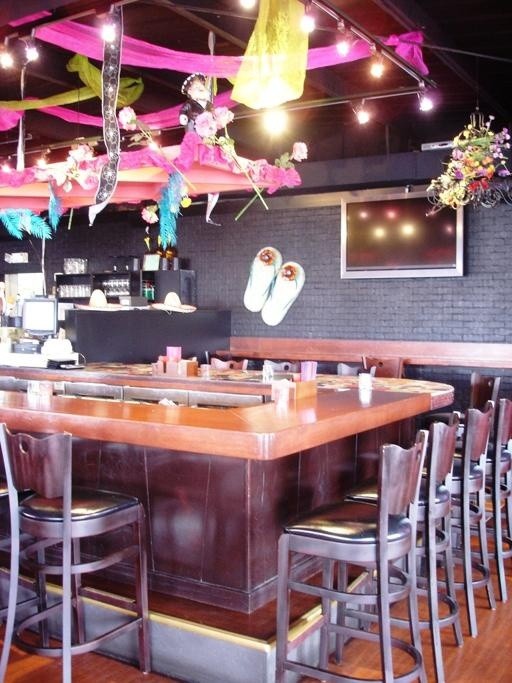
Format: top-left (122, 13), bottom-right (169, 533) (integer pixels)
top-left (335, 410), bottom-right (467, 683)
top-left (470, 371), bottom-right (503, 413)
top-left (275, 427), bottom-right (432, 683)
top-left (0, 421), bottom-right (155, 683)
top-left (362, 355), bottom-right (404, 379)
top-left (449, 396), bottom-right (512, 604)
top-left (417, 398), bottom-right (499, 640)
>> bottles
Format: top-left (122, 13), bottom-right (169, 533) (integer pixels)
top-left (143, 279), bottom-right (154, 301)
top-left (101, 278), bottom-right (130, 297)
top-left (57, 282), bottom-right (91, 300)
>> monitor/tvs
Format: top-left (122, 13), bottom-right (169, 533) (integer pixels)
top-left (20, 299), bottom-right (59, 335)
top-left (340, 189), bottom-right (464, 279)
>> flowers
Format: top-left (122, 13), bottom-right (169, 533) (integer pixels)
top-left (424, 107), bottom-right (511, 208)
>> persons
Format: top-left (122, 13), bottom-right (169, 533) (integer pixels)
top-left (181, 70), bottom-right (217, 130)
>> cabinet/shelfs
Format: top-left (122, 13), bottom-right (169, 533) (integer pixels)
top-left (55, 269), bottom-right (156, 332)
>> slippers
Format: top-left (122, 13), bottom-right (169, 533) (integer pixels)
top-left (262, 261), bottom-right (305, 325)
top-left (244, 247), bottom-right (282, 312)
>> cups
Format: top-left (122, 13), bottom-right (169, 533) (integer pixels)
top-left (57, 285), bottom-right (91, 297)
top-left (262, 365), bottom-right (273, 382)
top-left (102, 279), bottom-right (130, 295)
top-left (358, 373), bottom-right (372, 391)
